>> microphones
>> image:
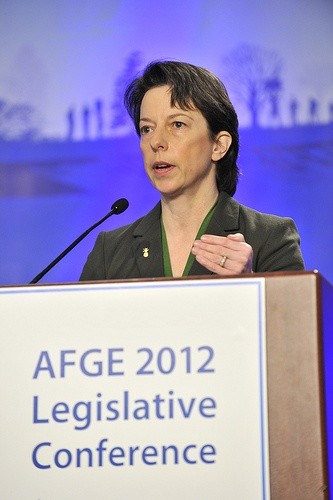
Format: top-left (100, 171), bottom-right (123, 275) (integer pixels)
top-left (29, 198), bottom-right (129, 284)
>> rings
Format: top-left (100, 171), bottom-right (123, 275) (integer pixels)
top-left (218, 254), bottom-right (231, 270)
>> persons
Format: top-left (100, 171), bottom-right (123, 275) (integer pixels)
top-left (79, 59), bottom-right (307, 280)
